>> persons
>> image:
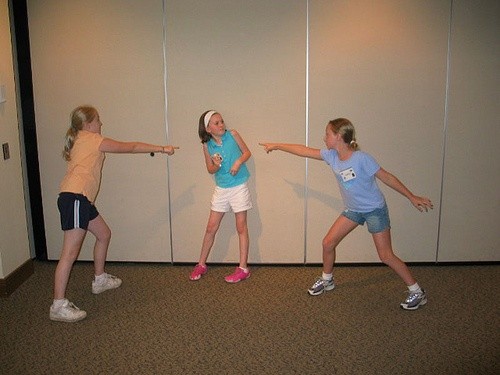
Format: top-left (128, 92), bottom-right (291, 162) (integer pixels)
top-left (49, 105), bottom-right (180, 323)
top-left (189, 111), bottom-right (252, 282)
top-left (259, 118), bottom-right (434, 310)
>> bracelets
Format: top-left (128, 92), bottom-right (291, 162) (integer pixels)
top-left (162, 146), bottom-right (164, 153)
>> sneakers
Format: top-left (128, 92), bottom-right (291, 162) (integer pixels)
top-left (48, 300), bottom-right (87, 322)
top-left (225, 267), bottom-right (251, 282)
top-left (92, 273), bottom-right (122, 294)
top-left (189, 264), bottom-right (208, 280)
top-left (400, 288), bottom-right (428, 311)
top-left (307, 275), bottom-right (336, 296)
